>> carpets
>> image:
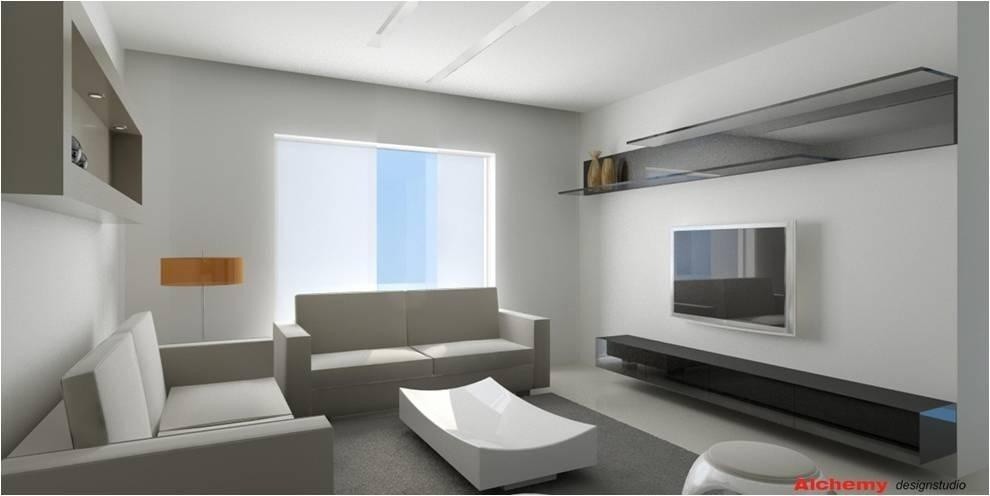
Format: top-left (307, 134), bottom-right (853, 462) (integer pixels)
top-left (330, 392), bottom-right (699, 495)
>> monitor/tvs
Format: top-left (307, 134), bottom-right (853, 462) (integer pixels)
top-left (669, 223), bottom-right (796, 337)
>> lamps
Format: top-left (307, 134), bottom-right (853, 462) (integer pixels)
top-left (160, 249), bottom-right (244, 342)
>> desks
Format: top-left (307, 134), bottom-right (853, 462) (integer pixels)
top-left (399, 376), bottom-right (597, 492)
top-left (595, 335), bottom-right (957, 467)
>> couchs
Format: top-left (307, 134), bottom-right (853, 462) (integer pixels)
top-left (273, 287), bottom-right (550, 418)
top-left (1, 311), bottom-right (333, 495)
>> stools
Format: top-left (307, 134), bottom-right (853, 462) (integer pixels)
top-left (682, 441), bottom-right (836, 495)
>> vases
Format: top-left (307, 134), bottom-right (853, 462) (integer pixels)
top-left (586, 151), bottom-right (601, 187)
top-left (601, 158), bottom-right (616, 184)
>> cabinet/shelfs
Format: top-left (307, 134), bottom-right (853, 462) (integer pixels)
top-left (1, 1), bottom-right (146, 225)
top-left (559, 66), bottom-right (957, 195)
